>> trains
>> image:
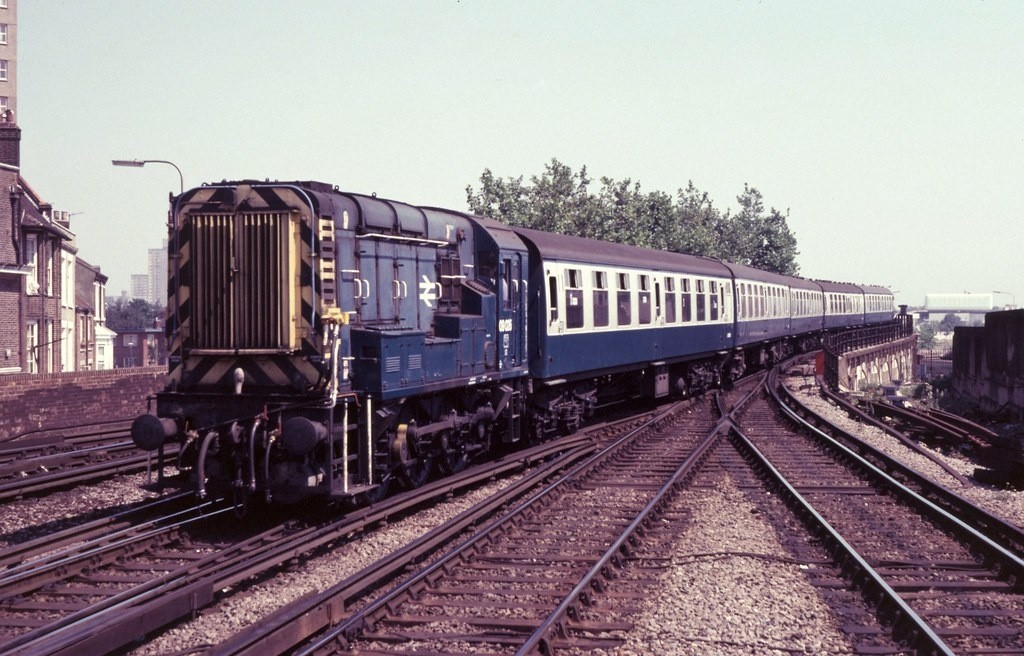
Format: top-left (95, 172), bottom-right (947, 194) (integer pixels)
top-left (130, 178), bottom-right (895, 519)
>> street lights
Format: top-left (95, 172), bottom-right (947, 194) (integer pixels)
top-left (112, 158), bottom-right (183, 193)
